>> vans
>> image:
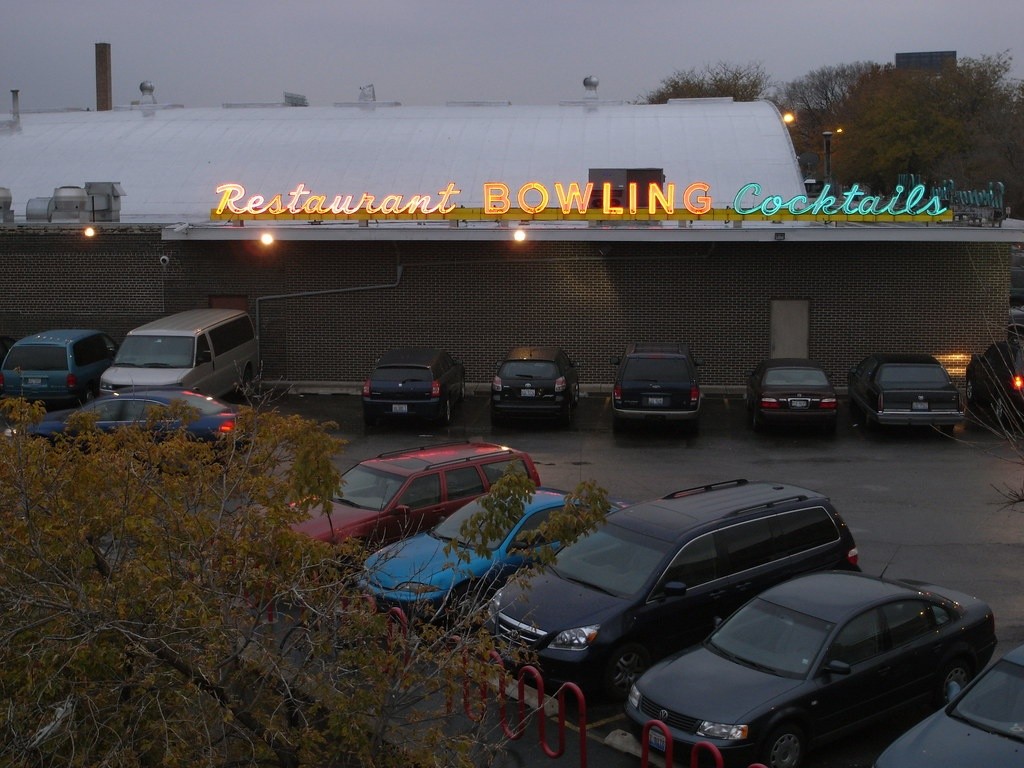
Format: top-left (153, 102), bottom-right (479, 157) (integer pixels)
top-left (98, 309), bottom-right (261, 412)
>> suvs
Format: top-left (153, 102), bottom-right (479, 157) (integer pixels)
top-left (272, 438), bottom-right (542, 566)
top-left (485, 476), bottom-right (863, 701)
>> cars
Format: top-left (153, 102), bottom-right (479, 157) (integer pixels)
top-left (361, 348), bottom-right (466, 431)
top-left (873, 643), bottom-right (1024, 768)
top-left (352, 486), bottom-right (633, 638)
top-left (748, 357), bottom-right (839, 436)
top-left (967, 340), bottom-right (1024, 429)
top-left (3, 391), bottom-right (258, 471)
top-left (620, 572), bottom-right (998, 767)
top-left (489, 346), bottom-right (581, 430)
top-left (0, 329), bottom-right (121, 409)
top-left (612, 339), bottom-right (707, 431)
top-left (848, 352), bottom-right (962, 435)
